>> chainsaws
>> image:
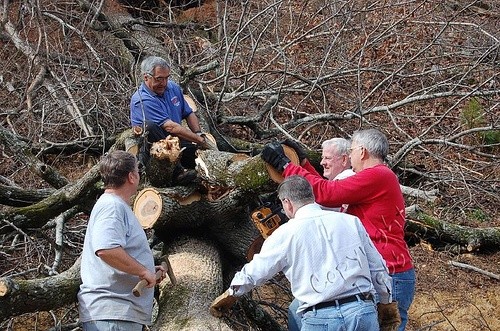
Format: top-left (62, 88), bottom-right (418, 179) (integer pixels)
top-left (248, 191), bottom-right (290, 240)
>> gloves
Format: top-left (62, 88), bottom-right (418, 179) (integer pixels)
top-left (377, 300), bottom-right (401, 331)
top-left (260, 143), bottom-right (291, 173)
top-left (283, 139), bottom-right (307, 163)
top-left (196, 131), bottom-right (219, 151)
top-left (210, 289), bottom-right (240, 319)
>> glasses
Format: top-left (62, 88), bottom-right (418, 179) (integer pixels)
top-left (147, 73), bottom-right (169, 82)
top-left (281, 199), bottom-right (286, 214)
top-left (347, 145), bottom-right (363, 155)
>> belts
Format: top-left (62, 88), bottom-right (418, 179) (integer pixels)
top-left (305, 292), bottom-right (374, 313)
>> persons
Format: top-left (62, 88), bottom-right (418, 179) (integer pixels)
top-left (317, 138), bottom-right (356, 212)
top-left (213, 174), bottom-right (402, 331)
top-left (77, 150), bottom-right (165, 331)
top-left (130, 56), bottom-right (205, 170)
top-left (260, 129), bottom-right (416, 331)
